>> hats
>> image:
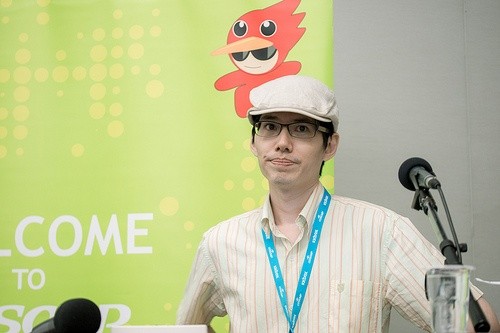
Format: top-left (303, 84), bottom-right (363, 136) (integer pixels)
top-left (247, 76), bottom-right (338, 132)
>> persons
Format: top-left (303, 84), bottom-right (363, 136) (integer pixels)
top-left (178, 74), bottom-right (500, 333)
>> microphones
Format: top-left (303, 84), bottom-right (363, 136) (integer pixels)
top-left (398, 157), bottom-right (441, 191)
top-left (30, 299), bottom-right (101, 333)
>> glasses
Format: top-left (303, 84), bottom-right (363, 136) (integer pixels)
top-left (254, 121), bottom-right (333, 139)
